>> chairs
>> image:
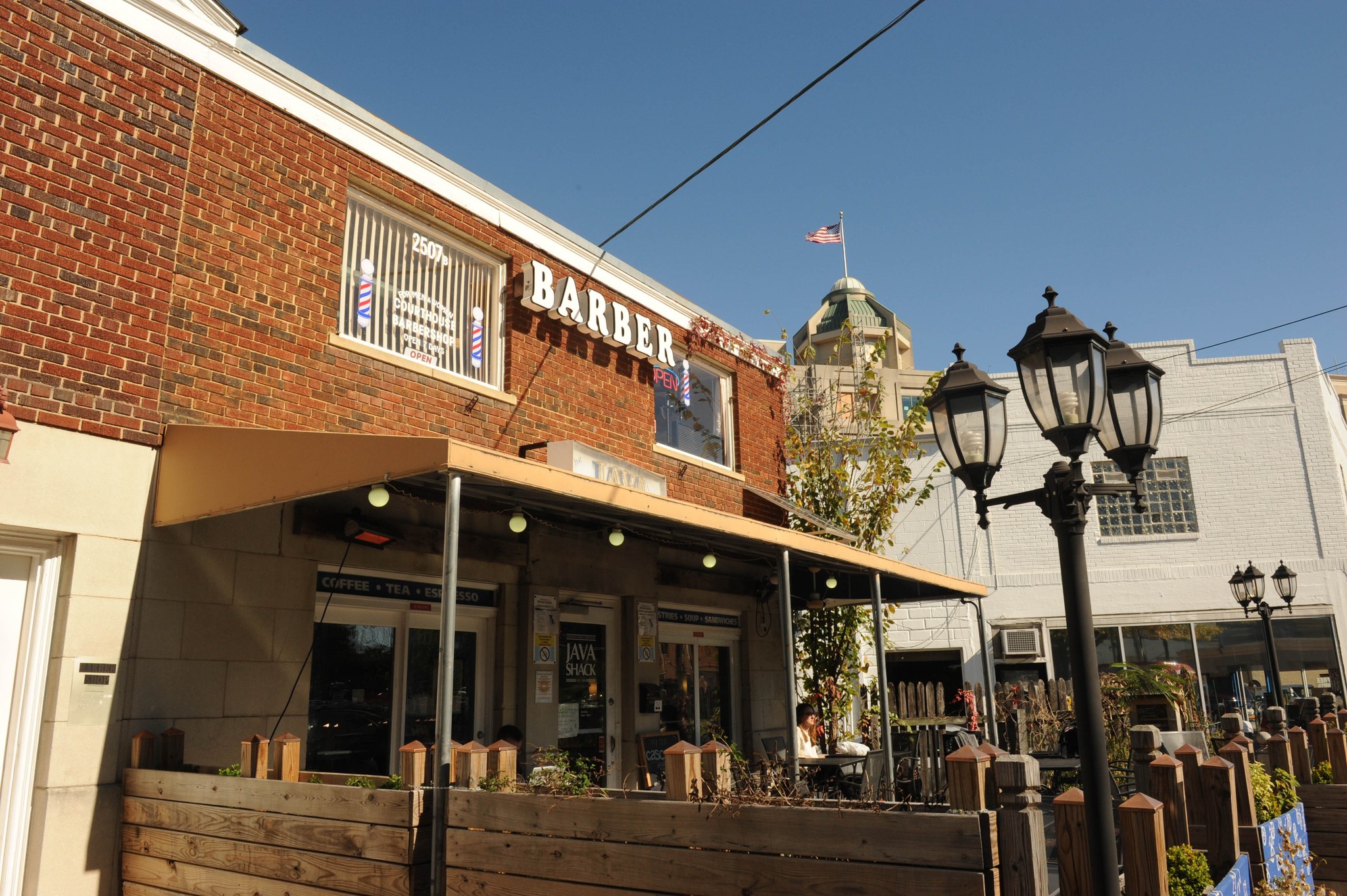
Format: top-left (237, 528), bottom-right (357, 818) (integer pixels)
top-left (730, 731), bottom-right (1136, 873)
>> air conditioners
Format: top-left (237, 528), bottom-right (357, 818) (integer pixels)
top-left (999, 627), bottom-right (1040, 656)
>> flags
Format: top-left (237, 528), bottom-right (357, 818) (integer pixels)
top-left (805, 220), bottom-right (841, 244)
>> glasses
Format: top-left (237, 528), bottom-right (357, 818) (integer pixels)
top-left (801, 712), bottom-right (815, 717)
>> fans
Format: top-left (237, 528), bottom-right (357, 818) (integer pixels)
top-left (774, 565), bottom-right (887, 609)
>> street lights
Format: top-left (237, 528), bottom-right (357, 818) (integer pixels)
top-left (919, 285), bottom-right (1168, 894)
top-left (1228, 559), bottom-right (1299, 732)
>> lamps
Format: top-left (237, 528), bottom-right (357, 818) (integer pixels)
top-left (769, 569), bottom-right (778, 585)
top-left (703, 549), bottom-right (716, 568)
top-left (609, 524), bottom-right (624, 546)
top-left (509, 508), bottom-right (527, 534)
top-left (368, 484), bottom-right (389, 507)
top-left (826, 571), bottom-right (837, 588)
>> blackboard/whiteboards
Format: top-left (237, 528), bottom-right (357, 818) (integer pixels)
top-left (638, 730), bottom-right (681, 787)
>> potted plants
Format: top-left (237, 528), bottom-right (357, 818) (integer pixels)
top-left (1047, 709), bottom-right (1347, 896)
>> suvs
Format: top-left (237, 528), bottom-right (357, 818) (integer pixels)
top-left (1238, 682), bottom-right (1317, 725)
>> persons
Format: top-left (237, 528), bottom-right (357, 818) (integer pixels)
top-left (497, 724), bottom-right (528, 784)
top-left (796, 703), bottom-right (845, 801)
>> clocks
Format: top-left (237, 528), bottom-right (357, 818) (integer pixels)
top-left (860, 341), bottom-right (880, 362)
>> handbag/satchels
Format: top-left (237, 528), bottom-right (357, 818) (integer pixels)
top-left (836, 742), bottom-right (870, 756)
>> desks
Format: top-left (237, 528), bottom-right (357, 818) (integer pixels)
top-left (797, 755), bottom-right (866, 803)
top-left (892, 751), bottom-right (910, 758)
top-left (1031, 755), bottom-right (1081, 796)
